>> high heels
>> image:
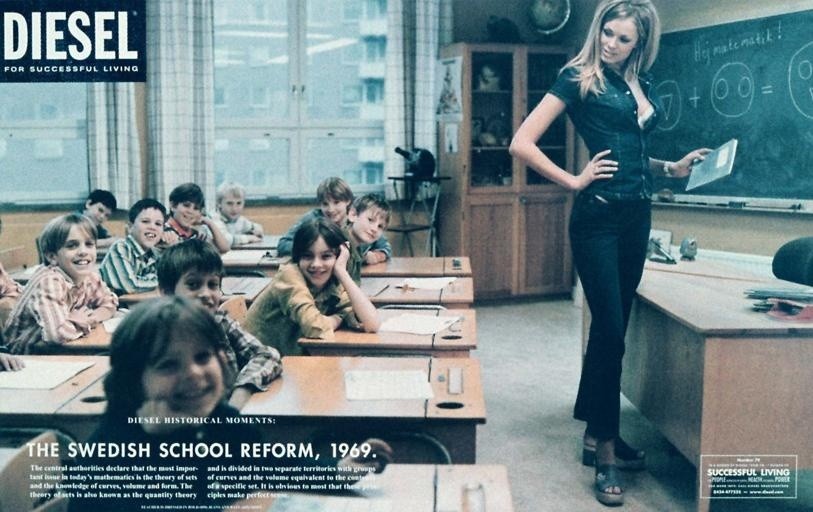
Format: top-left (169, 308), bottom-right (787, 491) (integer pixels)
top-left (583, 437), bottom-right (648, 470)
top-left (609, 486), bottom-right (611, 487)
top-left (594, 455), bottom-right (624, 505)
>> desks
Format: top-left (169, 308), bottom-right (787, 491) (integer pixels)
top-left (0, 233), bottom-right (517, 511)
top-left (577, 253), bottom-right (812, 512)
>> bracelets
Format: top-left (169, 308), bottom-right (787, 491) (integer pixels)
top-left (663, 161), bottom-right (673, 179)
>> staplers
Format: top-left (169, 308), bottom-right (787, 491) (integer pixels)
top-left (650, 239), bottom-right (678, 264)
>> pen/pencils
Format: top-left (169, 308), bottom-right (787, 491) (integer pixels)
top-left (677, 201), bottom-right (689, 204)
top-left (696, 202), bottom-right (707, 205)
top-left (716, 203), bottom-right (728, 206)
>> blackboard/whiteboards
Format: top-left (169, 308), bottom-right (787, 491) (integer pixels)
top-left (639, 9), bottom-right (813, 214)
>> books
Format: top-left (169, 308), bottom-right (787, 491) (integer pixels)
top-left (751, 297), bottom-right (812, 312)
top-left (742, 283), bottom-right (812, 299)
top-left (683, 138), bottom-right (738, 193)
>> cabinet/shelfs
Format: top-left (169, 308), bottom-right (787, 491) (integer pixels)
top-left (433, 40), bottom-right (579, 299)
top-left (385, 174), bottom-right (453, 258)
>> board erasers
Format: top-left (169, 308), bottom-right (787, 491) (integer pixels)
top-left (729, 201), bottom-right (746, 209)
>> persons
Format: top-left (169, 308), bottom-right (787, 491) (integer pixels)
top-left (159, 182), bottom-right (230, 259)
top-left (98, 198), bottom-right (167, 300)
top-left (75, 187), bottom-right (120, 250)
top-left (0, 217), bottom-right (26, 374)
top-left (71, 294), bottom-right (395, 512)
top-left (3, 211), bottom-right (119, 355)
top-left (238, 216), bottom-right (386, 359)
top-left (207, 177), bottom-right (264, 247)
top-left (507, 2), bottom-right (715, 506)
top-left (276, 174), bottom-right (394, 266)
top-left (151, 236), bottom-right (285, 411)
top-left (343, 191), bottom-right (391, 250)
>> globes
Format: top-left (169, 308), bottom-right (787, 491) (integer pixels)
top-left (530, 0), bottom-right (571, 44)
